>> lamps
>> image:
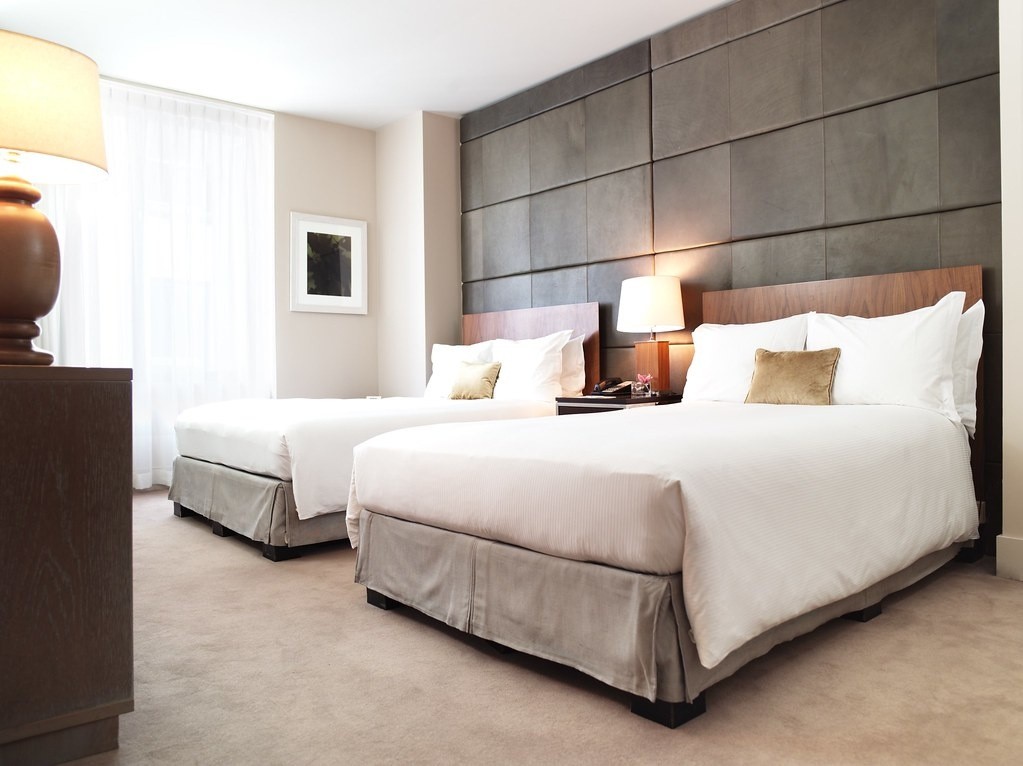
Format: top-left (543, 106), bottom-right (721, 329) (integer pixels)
top-left (616, 276), bottom-right (686, 391)
top-left (0, 28), bottom-right (108, 364)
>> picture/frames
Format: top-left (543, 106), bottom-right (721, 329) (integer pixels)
top-left (289, 211), bottom-right (368, 315)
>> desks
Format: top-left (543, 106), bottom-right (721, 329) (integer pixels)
top-left (0, 364), bottom-right (136, 766)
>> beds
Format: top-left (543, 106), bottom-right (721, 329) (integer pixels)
top-left (166, 302), bottom-right (598, 563)
top-left (347, 265), bottom-right (987, 729)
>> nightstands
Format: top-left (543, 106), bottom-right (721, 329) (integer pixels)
top-left (555, 391), bottom-right (683, 416)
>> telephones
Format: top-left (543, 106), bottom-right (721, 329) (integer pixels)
top-left (590, 377), bottom-right (634, 396)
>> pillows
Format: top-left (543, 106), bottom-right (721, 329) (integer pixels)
top-left (426, 329), bottom-right (588, 399)
top-left (681, 290), bottom-right (985, 436)
